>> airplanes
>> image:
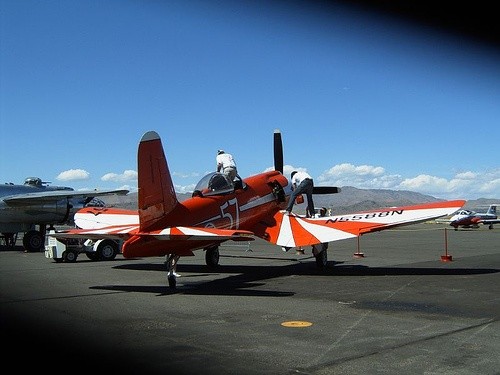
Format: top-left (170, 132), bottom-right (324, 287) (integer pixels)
top-left (448, 205), bottom-right (500, 230)
top-left (45, 206), bottom-right (140, 263)
top-left (0, 176), bottom-right (130, 252)
top-left (121, 129), bottom-right (466, 290)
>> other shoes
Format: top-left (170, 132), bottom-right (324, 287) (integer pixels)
top-left (311, 215), bottom-right (315, 218)
top-left (285, 211), bottom-right (290, 215)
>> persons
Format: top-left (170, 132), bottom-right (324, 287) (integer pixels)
top-left (165, 254), bottom-right (182, 277)
top-left (2, 233), bottom-right (15, 248)
top-left (313, 207), bottom-right (328, 270)
top-left (286, 172), bottom-right (315, 218)
top-left (216, 149), bottom-right (237, 185)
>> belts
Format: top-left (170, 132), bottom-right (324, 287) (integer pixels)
top-left (226, 166), bottom-right (236, 169)
